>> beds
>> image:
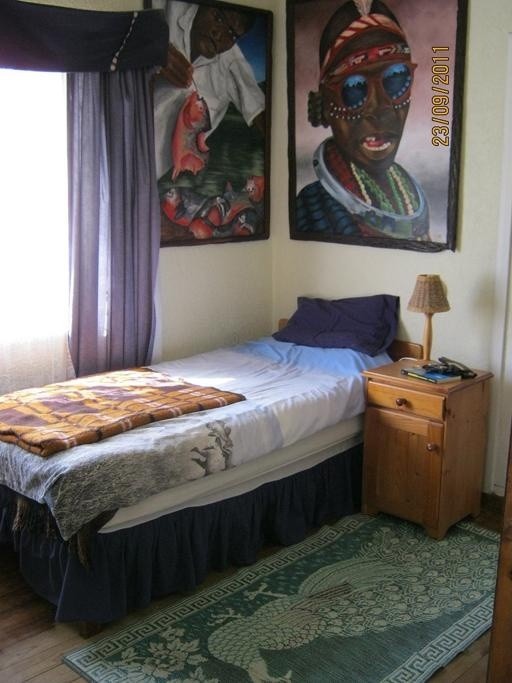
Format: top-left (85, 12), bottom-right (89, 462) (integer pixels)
top-left (0, 319), bottom-right (422, 639)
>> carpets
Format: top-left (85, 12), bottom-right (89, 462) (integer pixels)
top-left (60, 513), bottom-right (502, 683)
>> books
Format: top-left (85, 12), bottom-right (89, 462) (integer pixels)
top-left (399, 367), bottom-right (461, 385)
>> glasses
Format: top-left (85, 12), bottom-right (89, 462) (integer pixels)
top-left (326, 63), bottom-right (417, 111)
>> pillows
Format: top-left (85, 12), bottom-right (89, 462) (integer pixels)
top-left (272, 294), bottom-right (400, 357)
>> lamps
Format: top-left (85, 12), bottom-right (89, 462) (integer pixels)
top-left (407, 274), bottom-right (450, 368)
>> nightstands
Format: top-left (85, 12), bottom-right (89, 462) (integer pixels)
top-left (359, 357), bottom-right (494, 541)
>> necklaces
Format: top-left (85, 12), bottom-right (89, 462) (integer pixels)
top-left (308, 138), bottom-right (425, 240)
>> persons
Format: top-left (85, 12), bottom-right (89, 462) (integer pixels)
top-left (298, 2), bottom-right (430, 243)
top-left (154, 4), bottom-right (267, 181)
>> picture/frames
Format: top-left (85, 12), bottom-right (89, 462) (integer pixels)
top-left (286, 0), bottom-right (468, 252)
top-left (142, 0), bottom-right (273, 248)
